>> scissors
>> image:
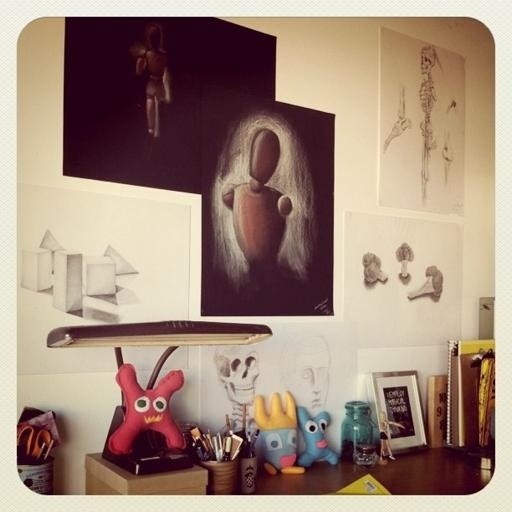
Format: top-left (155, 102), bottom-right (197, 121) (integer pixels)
top-left (18, 427), bottom-right (51, 459)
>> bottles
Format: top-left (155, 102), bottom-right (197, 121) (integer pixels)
top-left (341, 401), bottom-right (373, 463)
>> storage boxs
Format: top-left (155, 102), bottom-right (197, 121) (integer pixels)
top-left (84, 453), bottom-right (209, 495)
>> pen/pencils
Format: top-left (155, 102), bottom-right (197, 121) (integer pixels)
top-left (190, 402), bottom-right (260, 461)
top-left (35, 439), bottom-right (56, 466)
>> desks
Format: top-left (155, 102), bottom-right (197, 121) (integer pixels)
top-left (205, 446), bottom-right (495, 495)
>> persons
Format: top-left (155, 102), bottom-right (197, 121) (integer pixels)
top-left (376, 410), bottom-right (406, 465)
top-left (135, 24), bottom-right (171, 138)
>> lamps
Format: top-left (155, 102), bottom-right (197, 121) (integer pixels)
top-left (47, 321), bottom-right (272, 475)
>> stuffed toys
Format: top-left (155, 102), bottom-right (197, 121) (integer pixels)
top-left (106, 362), bottom-right (188, 456)
top-left (294, 403), bottom-right (339, 468)
top-left (252, 389), bottom-right (307, 477)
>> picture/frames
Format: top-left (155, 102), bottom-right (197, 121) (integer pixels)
top-left (371, 370), bottom-right (429, 457)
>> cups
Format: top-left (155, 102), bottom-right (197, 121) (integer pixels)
top-left (353, 424), bottom-right (377, 467)
top-left (15, 454), bottom-right (54, 495)
top-left (201, 456), bottom-right (258, 495)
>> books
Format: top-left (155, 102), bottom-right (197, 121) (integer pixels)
top-left (425, 338), bottom-right (494, 452)
top-left (335, 473), bottom-right (391, 495)
